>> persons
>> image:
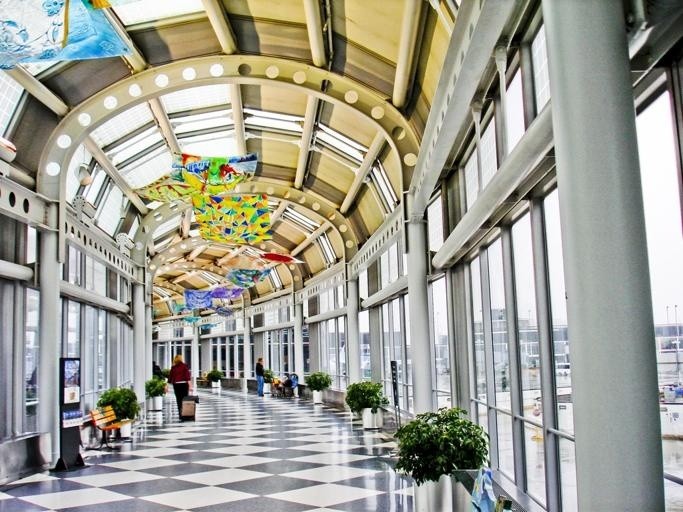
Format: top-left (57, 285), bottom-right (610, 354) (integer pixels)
top-left (274, 372), bottom-right (293, 397)
top-left (152, 360), bottom-right (163, 397)
top-left (255, 357), bottom-right (265, 397)
top-left (167, 355), bottom-right (193, 419)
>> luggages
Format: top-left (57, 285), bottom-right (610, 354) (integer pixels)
top-left (180, 388), bottom-right (198, 421)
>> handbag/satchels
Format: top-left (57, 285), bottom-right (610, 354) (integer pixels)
top-left (183, 395), bottom-right (199, 403)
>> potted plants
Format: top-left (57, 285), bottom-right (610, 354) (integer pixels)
top-left (345, 381), bottom-right (389, 429)
top-left (392, 406), bottom-right (489, 511)
top-left (206, 368), bottom-right (224, 388)
top-left (144, 378), bottom-right (166, 412)
top-left (304, 371), bottom-right (332, 404)
top-left (96, 386), bottom-right (140, 438)
top-left (261, 368), bottom-right (273, 394)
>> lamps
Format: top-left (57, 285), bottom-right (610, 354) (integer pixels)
top-left (72, 145), bottom-right (92, 186)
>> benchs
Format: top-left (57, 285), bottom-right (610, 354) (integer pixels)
top-left (90, 404), bottom-right (137, 451)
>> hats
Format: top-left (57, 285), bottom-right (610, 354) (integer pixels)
top-left (173, 355), bottom-right (185, 362)
